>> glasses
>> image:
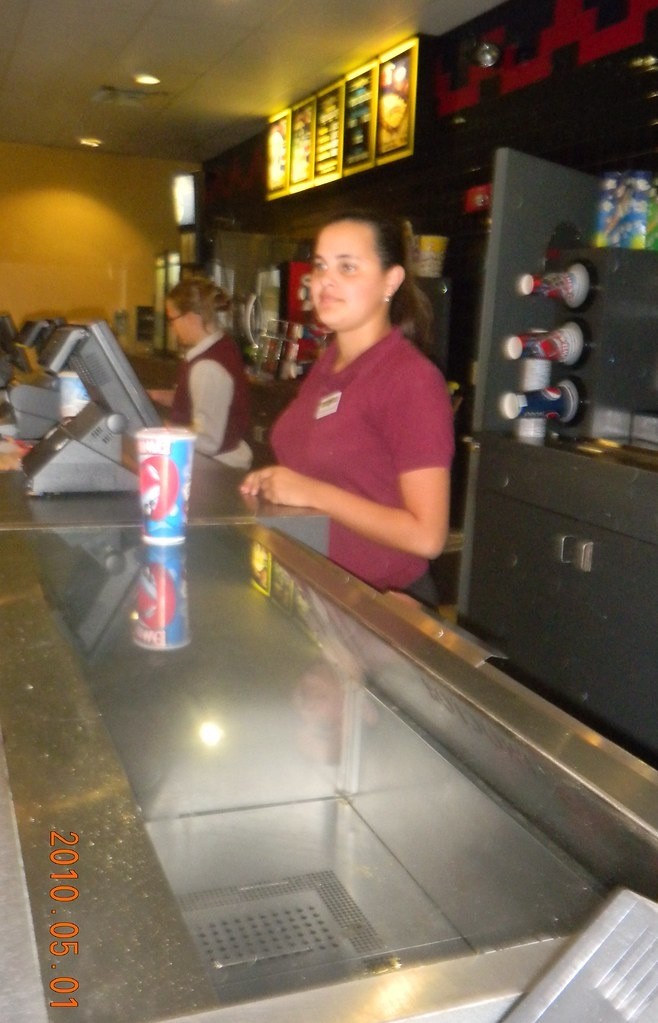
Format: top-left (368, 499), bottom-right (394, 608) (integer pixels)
top-left (165, 311), bottom-right (190, 327)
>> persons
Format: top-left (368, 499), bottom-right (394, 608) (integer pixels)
top-left (239, 208), bottom-right (457, 613)
top-left (144, 276), bottom-right (255, 474)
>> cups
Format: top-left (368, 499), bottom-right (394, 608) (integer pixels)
top-left (520, 271), bottom-right (580, 304)
top-left (135, 428), bottom-right (197, 547)
top-left (506, 328), bottom-right (574, 360)
top-left (133, 543), bottom-right (194, 652)
top-left (58, 371), bottom-right (92, 419)
top-left (504, 383), bottom-right (574, 421)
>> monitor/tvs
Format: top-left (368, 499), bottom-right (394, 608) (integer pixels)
top-left (0, 310), bottom-right (164, 437)
